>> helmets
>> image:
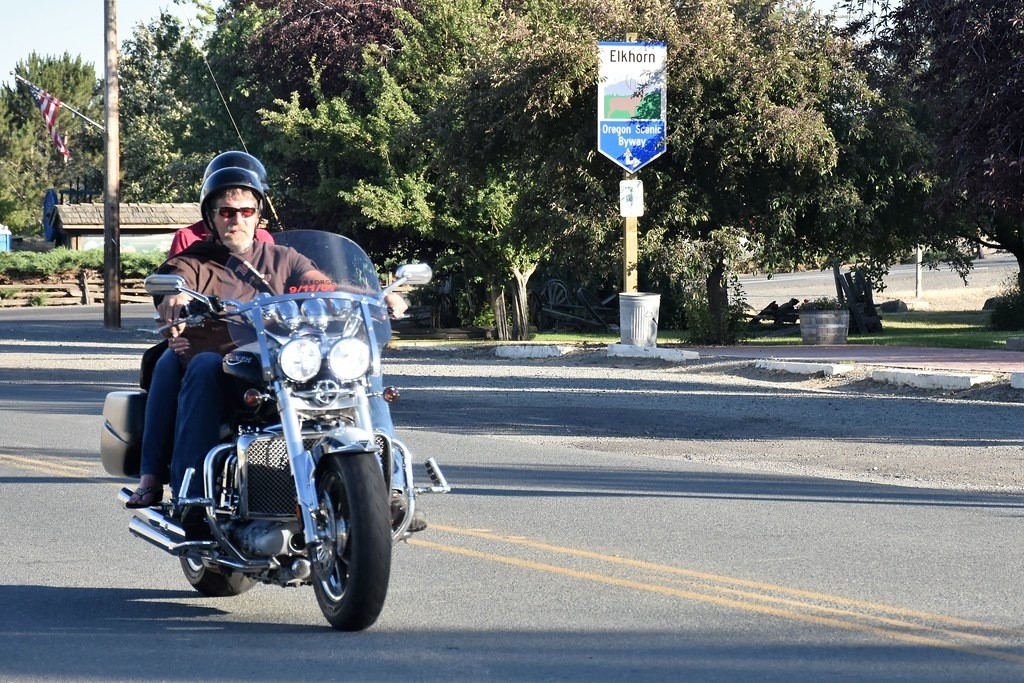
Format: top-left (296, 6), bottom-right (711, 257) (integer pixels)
top-left (199, 167), bottom-right (265, 217)
top-left (203, 151), bottom-right (270, 190)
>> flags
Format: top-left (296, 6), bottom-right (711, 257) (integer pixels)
top-left (16, 77), bottom-right (70, 157)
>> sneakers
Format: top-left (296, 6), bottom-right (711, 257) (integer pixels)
top-left (180, 504), bottom-right (207, 540)
top-left (391, 490), bottom-right (426, 532)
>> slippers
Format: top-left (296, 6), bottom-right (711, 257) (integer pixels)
top-left (126, 485), bottom-right (163, 508)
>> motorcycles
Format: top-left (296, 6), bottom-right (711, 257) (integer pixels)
top-left (100, 229), bottom-right (452, 633)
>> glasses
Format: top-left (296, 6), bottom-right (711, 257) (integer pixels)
top-left (213, 207), bottom-right (259, 218)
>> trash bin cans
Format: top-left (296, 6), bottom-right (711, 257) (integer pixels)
top-left (619, 292), bottom-right (662, 348)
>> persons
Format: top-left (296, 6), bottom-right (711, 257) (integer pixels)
top-left (154, 166), bottom-right (425, 544)
top-left (125, 151), bottom-right (274, 510)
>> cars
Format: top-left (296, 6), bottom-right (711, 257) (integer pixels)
top-left (375, 269), bottom-right (477, 329)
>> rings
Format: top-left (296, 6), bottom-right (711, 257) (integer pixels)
top-left (175, 349), bottom-right (177, 352)
top-left (172, 338), bottom-right (175, 342)
